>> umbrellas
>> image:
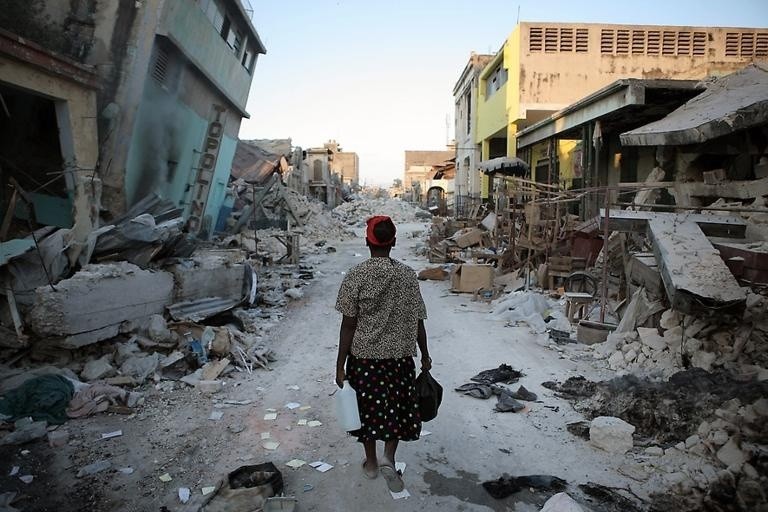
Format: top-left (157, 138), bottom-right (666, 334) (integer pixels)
top-left (478, 157), bottom-right (529, 184)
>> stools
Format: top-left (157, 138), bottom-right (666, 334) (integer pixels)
top-left (562, 292), bottom-right (593, 325)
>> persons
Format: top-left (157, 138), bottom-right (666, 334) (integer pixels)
top-left (334, 216), bottom-right (432, 492)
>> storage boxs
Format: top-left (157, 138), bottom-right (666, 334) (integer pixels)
top-left (449, 262), bottom-right (495, 294)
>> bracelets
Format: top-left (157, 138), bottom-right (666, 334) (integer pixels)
top-left (422, 357), bottom-right (432, 365)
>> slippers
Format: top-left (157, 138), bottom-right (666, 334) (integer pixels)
top-left (361, 457), bottom-right (378, 480)
top-left (380, 466), bottom-right (403, 493)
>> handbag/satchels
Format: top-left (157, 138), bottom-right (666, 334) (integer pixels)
top-left (414, 371), bottom-right (443, 422)
